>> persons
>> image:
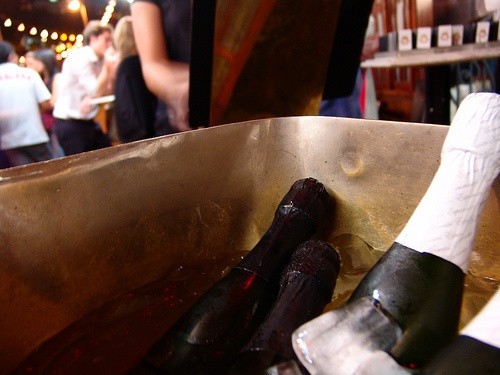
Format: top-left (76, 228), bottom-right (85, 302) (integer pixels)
top-left (50, 21), bottom-right (114, 155)
top-left (24, 49), bottom-right (64, 160)
top-left (127, 0), bottom-right (376, 139)
top-left (104, 15), bottom-right (157, 145)
top-left (0, 43), bottom-right (52, 167)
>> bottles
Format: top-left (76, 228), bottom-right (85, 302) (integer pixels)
top-left (135, 177), bottom-right (334, 374)
top-left (415, 288), bottom-right (500, 375)
top-left (294, 92), bottom-right (500, 375)
top-left (231, 239), bottom-right (340, 375)
